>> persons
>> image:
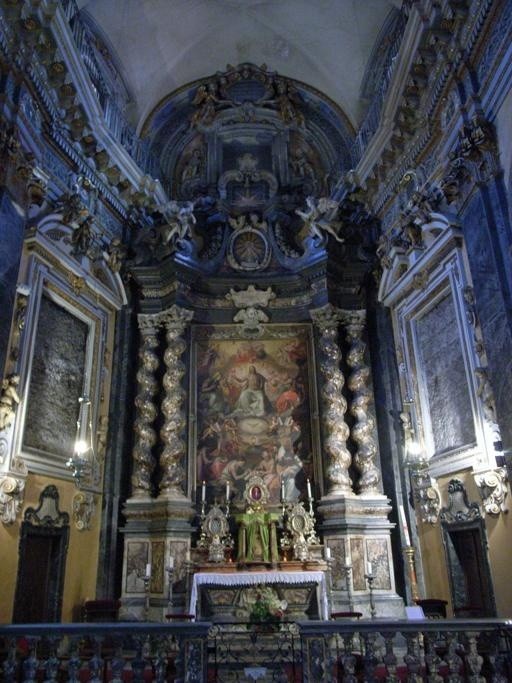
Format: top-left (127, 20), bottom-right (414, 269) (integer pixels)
top-left (198, 339), bottom-right (314, 505)
top-left (176, 200), bottom-right (197, 239)
top-left (294, 196), bottom-right (346, 243)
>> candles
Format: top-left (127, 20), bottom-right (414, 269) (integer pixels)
top-left (399, 505), bottom-right (410, 545)
top-left (146, 556), bottom-right (173, 577)
top-left (326, 546), bottom-right (372, 574)
top-left (201, 478), bottom-right (312, 500)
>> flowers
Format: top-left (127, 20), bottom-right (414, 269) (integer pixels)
top-left (246, 587), bottom-right (288, 635)
